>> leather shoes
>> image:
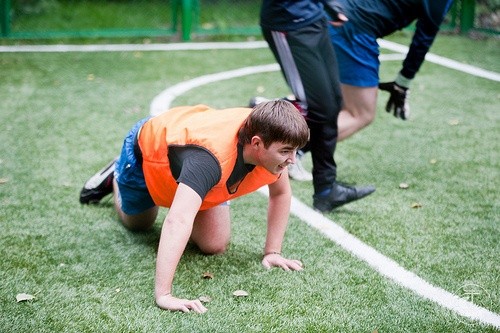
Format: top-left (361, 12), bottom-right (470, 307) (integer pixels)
top-left (312, 181), bottom-right (376, 211)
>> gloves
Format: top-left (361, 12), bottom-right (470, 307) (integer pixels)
top-left (376, 81), bottom-right (409, 121)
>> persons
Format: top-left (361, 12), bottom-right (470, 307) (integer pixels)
top-left (80, 100), bottom-right (309, 313)
top-left (246, 0), bottom-right (452, 212)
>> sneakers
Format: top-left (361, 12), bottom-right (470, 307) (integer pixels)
top-left (289, 157), bottom-right (312, 180)
top-left (80, 154), bottom-right (116, 203)
